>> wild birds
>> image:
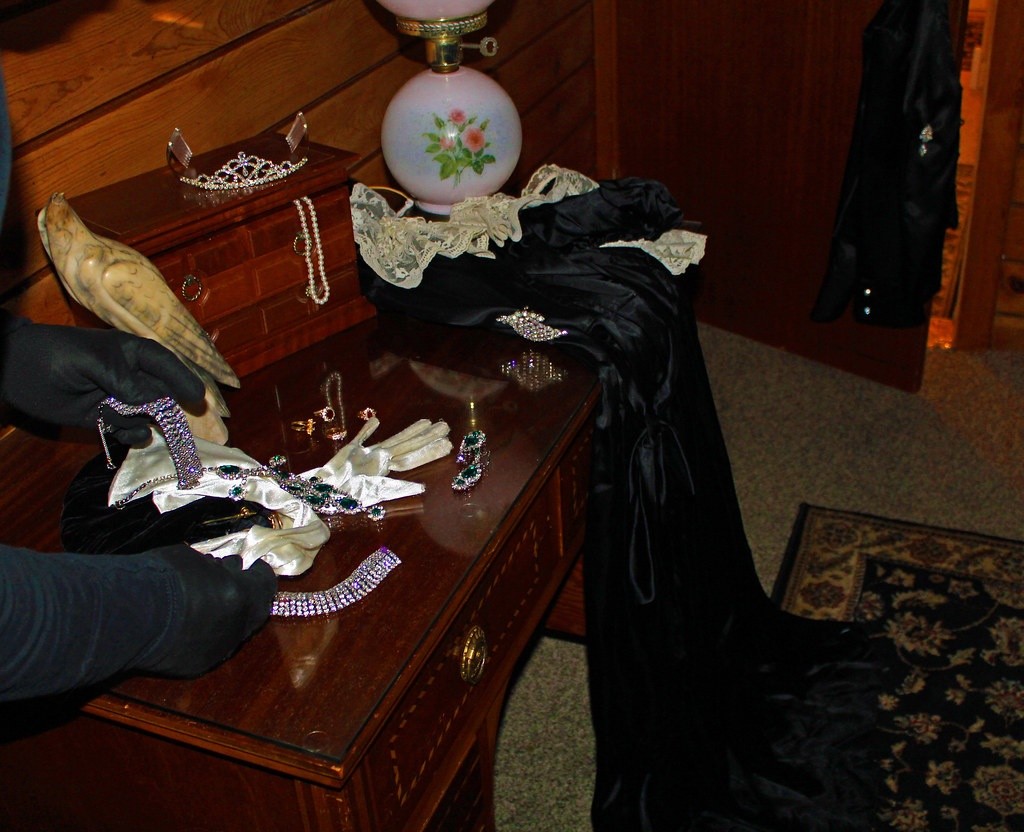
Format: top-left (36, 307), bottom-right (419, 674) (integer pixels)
top-left (37, 191), bottom-right (242, 418)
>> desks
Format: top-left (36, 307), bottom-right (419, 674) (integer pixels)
top-left (0, 315), bottom-right (602, 832)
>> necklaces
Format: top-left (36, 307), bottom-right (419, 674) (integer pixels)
top-left (110, 455), bottom-right (387, 522)
top-left (293, 196), bottom-right (331, 306)
top-left (324, 372), bottom-right (346, 430)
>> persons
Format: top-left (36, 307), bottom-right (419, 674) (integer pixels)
top-left (0, 295), bottom-right (276, 705)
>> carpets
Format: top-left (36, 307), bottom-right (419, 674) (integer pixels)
top-left (770, 503), bottom-right (1024, 832)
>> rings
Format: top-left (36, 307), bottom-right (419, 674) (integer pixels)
top-left (290, 418), bottom-right (316, 436)
top-left (358, 407), bottom-right (376, 421)
top-left (313, 406), bottom-right (335, 422)
top-left (324, 427), bottom-right (347, 442)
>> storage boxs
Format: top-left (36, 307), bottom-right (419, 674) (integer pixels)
top-left (36, 131), bottom-right (379, 380)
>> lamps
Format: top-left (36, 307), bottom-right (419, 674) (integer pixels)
top-left (377, 0), bottom-right (523, 215)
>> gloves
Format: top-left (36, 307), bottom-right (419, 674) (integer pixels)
top-left (285, 416), bottom-right (454, 517)
top-left (142, 543), bottom-right (278, 680)
top-left (1, 310), bottom-right (205, 446)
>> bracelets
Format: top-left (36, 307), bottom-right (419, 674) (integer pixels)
top-left (96, 397), bottom-right (203, 490)
top-left (270, 546), bottom-right (402, 617)
